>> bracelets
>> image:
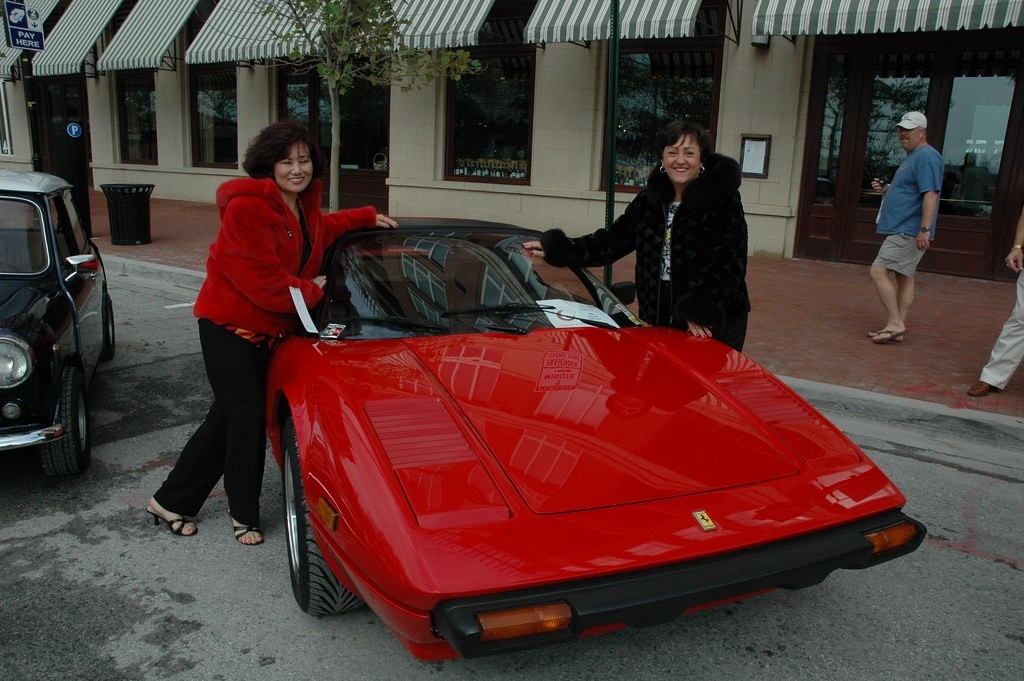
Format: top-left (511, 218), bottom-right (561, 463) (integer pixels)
top-left (1012, 244), bottom-right (1023, 249)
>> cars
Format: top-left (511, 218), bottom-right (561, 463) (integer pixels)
top-left (0, 169), bottom-right (116, 478)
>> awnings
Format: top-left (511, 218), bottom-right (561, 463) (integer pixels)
top-left (0, 0), bottom-right (61, 81)
top-left (96, 0), bottom-right (200, 72)
top-left (522, 0), bottom-right (743, 46)
top-left (31, 0), bottom-right (124, 79)
top-left (752, 0), bottom-right (1024, 45)
top-left (349, 0), bottom-right (533, 53)
top-left (184, 0), bottom-right (329, 65)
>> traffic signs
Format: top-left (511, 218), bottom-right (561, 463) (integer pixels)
top-left (2, 0), bottom-right (44, 51)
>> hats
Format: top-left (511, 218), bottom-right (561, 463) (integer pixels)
top-left (894, 111), bottom-right (927, 129)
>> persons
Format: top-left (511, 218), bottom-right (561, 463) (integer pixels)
top-left (966, 204), bottom-right (1024, 396)
top-left (522, 118), bottom-right (751, 353)
top-left (146, 120), bottom-right (400, 546)
top-left (864, 111), bottom-right (945, 342)
top-left (958, 151), bottom-right (989, 216)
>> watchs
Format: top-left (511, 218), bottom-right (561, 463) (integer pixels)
top-left (921, 226), bottom-right (930, 232)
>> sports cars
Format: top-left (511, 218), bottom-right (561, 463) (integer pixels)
top-left (266, 215), bottom-right (928, 661)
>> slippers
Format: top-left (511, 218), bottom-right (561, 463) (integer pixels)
top-left (864, 330), bottom-right (904, 343)
top-left (872, 329), bottom-right (907, 343)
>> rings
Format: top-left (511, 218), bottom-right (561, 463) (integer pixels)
top-left (315, 279), bottom-right (320, 283)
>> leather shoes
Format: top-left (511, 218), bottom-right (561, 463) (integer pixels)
top-left (967, 381), bottom-right (1002, 396)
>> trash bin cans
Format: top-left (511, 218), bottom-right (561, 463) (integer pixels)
top-left (99, 182), bottom-right (156, 246)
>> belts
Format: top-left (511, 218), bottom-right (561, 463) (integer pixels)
top-left (209, 318), bottom-right (279, 353)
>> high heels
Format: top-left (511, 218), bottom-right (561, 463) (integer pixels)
top-left (146, 505), bottom-right (198, 537)
top-left (227, 507), bottom-right (264, 545)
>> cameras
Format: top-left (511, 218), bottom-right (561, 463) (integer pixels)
top-left (877, 176), bottom-right (888, 187)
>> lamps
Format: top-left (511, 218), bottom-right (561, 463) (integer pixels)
top-left (751, 33), bottom-right (771, 48)
